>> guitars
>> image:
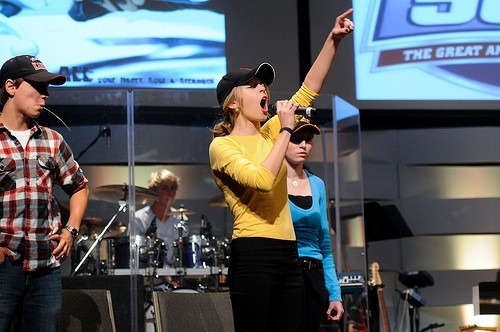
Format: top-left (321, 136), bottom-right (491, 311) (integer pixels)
top-left (369, 262), bottom-right (391, 332)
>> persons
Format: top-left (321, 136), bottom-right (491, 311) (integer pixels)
top-left (0, 55), bottom-right (89, 332)
top-left (284, 117), bottom-right (345, 332)
top-left (127, 169), bottom-right (190, 263)
top-left (209, 8), bottom-right (354, 332)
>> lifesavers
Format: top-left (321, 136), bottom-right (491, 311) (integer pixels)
top-left (106, 236), bottom-right (166, 269)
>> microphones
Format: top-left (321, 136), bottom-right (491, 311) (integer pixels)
top-left (146, 217), bottom-right (157, 238)
top-left (200, 216), bottom-right (205, 235)
top-left (268, 103), bottom-right (317, 116)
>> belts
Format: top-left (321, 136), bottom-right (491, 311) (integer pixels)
top-left (299, 258), bottom-right (316, 269)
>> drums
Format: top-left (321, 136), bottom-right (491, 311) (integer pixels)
top-left (142, 289), bottom-right (203, 332)
top-left (221, 237), bottom-right (231, 267)
top-left (176, 237), bottom-right (220, 278)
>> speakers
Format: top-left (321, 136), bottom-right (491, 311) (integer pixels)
top-left (72, 236), bottom-right (147, 332)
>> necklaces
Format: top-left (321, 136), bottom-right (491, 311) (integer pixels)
top-left (287, 170), bottom-right (303, 187)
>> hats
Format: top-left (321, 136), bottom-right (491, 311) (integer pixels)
top-left (216, 62), bottom-right (276, 105)
top-left (0, 55), bottom-right (66, 86)
top-left (292, 114), bottom-right (321, 135)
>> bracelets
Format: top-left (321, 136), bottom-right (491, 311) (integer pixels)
top-left (63, 225), bottom-right (78, 239)
top-left (279, 126), bottom-right (293, 136)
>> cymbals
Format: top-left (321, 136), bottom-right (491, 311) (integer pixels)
top-left (165, 210), bottom-right (197, 214)
top-left (75, 217), bottom-right (127, 240)
top-left (208, 194), bottom-right (229, 207)
top-left (92, 185), bottom-right (160, 204)
